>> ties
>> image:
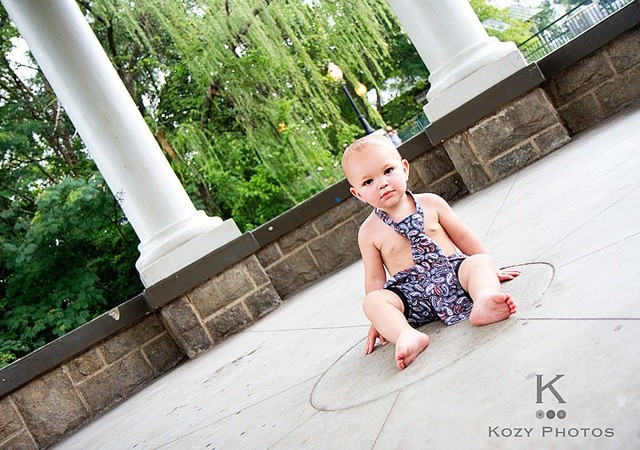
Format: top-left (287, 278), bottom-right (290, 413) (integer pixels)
top-left (375, 189), bottom-right (473, 326)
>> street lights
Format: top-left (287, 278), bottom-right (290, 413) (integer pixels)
top-left (327, 62), bottom-right (375, 134)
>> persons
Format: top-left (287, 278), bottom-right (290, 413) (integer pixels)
top-left (385, 126), bottom-right (401, 146)
top-left (340, 134), bottom-right (522, 371)
